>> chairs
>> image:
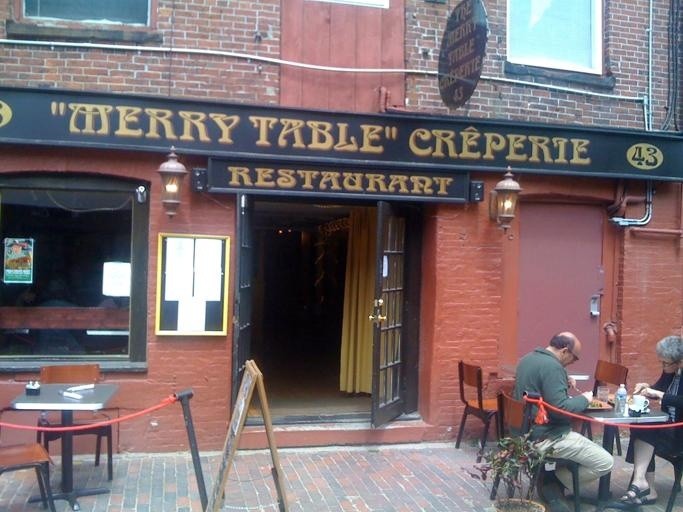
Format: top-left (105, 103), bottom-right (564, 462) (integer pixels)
top-left (641, 436), bottom-right (682, 512)
top-left (580, 360), bottom-right (629, 457)
top-left (489, 389), bottom-right (583, 512)
top-left (453, 359), bottom-right (499, 463)
top-left (0, 441), bottom-right (57, 512)
top-left (35, 364), bottom-right (114, 482)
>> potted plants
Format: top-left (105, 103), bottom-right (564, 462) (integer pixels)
top-left (460, 435), bottom-right (555, 512)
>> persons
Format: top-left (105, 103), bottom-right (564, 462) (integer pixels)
top-left (615, 335), bottom-right (683, 508)
top-left (506, 327), bottom-right (615, 507)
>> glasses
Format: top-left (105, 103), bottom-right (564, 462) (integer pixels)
top-left (663, 360), bottom-right (679, 367)
top-left (563, 345), bottom-right (579, 361)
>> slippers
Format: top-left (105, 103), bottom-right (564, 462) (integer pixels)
top-left (618, 485), bottom-right (649, 502)
top-left (623, 495), bottom-right (656, 505)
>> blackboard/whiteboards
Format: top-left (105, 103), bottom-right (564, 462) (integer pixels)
top-left (155, 231), bottom-right (231, 337)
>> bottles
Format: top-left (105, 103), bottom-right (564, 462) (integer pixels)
top-left (27, 380), bottom-right (39, 388)
top-left (613, 383), bottom-right (626, 415)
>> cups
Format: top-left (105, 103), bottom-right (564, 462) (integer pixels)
top-left (630, 394), bottom-right (649, 410)
top-left (596, 386), bottom-right (609, 403)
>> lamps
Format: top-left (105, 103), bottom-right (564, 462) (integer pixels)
top-left (488, 164), bottom-right (523, 242)
top-left (153, 143), bottom-right (189, 218)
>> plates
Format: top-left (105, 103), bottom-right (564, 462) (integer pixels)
top-left (629, 405), bottom-right (650, 413)
top-left (580, 399), bottom-right (612, 410)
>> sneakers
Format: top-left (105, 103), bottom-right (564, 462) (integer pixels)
top-left (533, 466), bottom-right (554, 502)
top-left (541, 482), bottom-right (571, 512)
